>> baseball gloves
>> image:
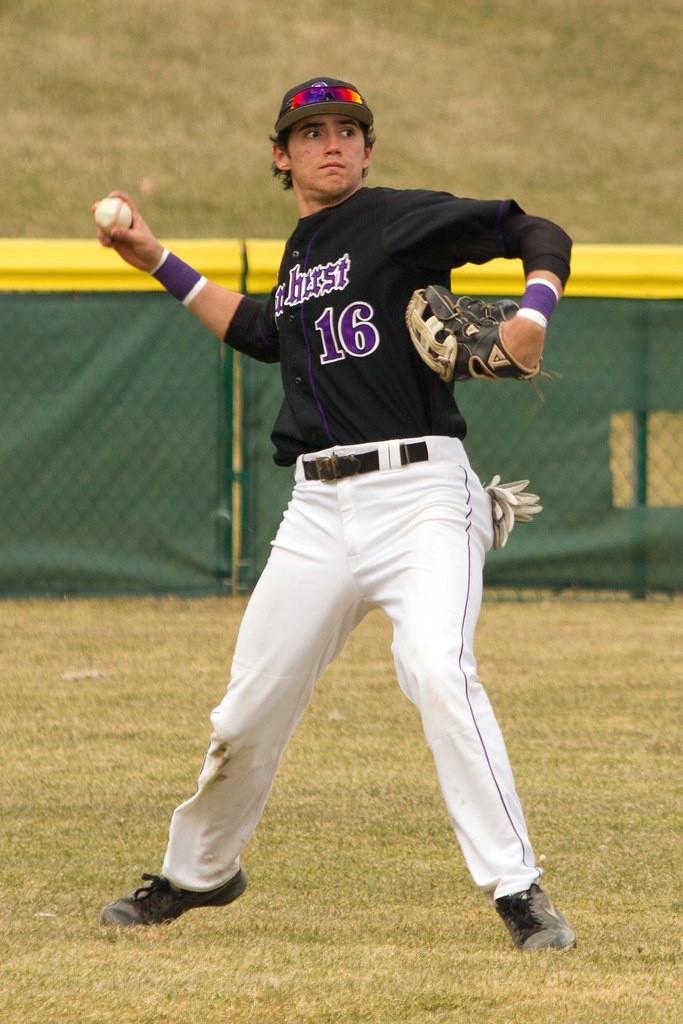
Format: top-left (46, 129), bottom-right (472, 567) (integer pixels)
top-left (405, 285), bottom-right (540, 382)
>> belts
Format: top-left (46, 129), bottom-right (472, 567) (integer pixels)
top-left (302, 441), bottom-right (429, 483)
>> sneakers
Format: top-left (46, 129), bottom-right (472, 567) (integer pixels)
top-left (495, 883), bottom-right (576, 954)
top-left (101, 865), bottom-right (245, 928)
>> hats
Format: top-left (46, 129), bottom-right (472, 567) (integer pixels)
top-left (273, 76), bottom-right (374, 133)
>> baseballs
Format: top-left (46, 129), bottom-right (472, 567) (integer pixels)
top-left (95, 197), bottom-right (133, 234)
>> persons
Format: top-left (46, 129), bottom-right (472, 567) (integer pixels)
top-left (90, 76), bottom-right (574, 955)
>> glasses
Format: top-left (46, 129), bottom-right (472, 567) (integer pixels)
top-left (283, 84), bottom-right (362, 110)
